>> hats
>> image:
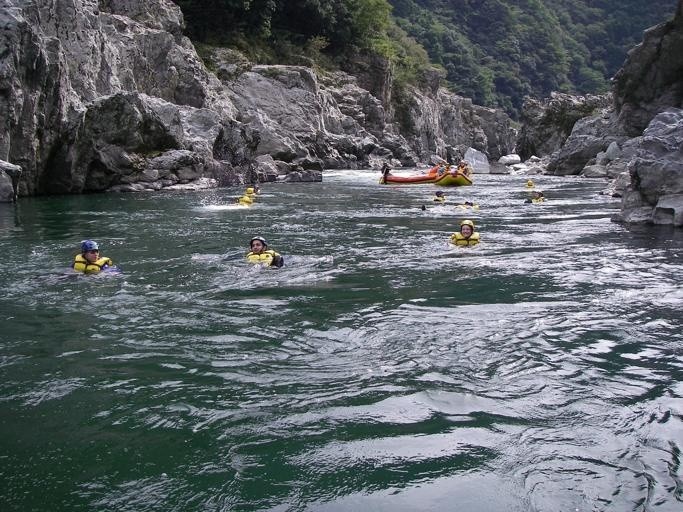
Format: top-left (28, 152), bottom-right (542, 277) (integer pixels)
top-left (436, 191), bottom-right (447, 197)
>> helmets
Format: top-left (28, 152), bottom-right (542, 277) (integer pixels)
top-left (438, 160), bottom-right (469, 168)
top-left (461, 219), bottom-right (474, 232)
top-left (250, 235), bottom-right (268, 249)
top-left (534, 191), bottom-right (544, 199)
top-left (81, 239), bottom-right (100, 253)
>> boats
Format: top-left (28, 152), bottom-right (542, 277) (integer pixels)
top-left (433, 169), bottom-right (473, 186)
top-left (379, 172), bottom-right (438, 184)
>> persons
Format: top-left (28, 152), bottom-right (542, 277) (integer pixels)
top-left (530, 190), bottom-right (548, 204)
top-left (431, 160), bottom-right (472, 178)
top-left (236, 187), bottom-right (262, 204)
top-left (433, 191), bottom-right (444, 200)
top-left (448, 220), bottom-right (480, 247)
top-left (66, 240), bottom-right (112, 275)
top-left (244, 235), bottom-right (283, 270)
top-left (380, 162), bottom-right (393, 176)
top-left (454, 200), bottom-right (479, 210)
